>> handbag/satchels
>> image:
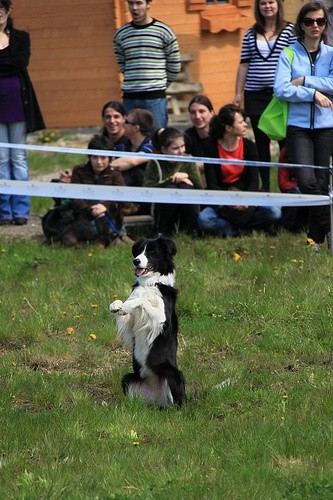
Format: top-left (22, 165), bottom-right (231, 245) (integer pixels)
top-left (258, 46), bottom-right (294, 141)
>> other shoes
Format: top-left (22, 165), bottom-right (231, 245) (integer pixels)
top-left (0, 218), bottom-right (12, 226)
top-left (306, 237), bottom-right (320, 253)
top-left (15, 217), bottom-right (28, 225)
top-left (327, 233), bottom-right (333, 248)
top-left (104, 234), bottom-right (135, 249)
top-left (192, 230), bottom-right (203, 240)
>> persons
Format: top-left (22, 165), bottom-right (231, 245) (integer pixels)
top-left (273, 1), bottom-right (333, 252)
top-left (0, 0), bottom-right (46, 225)
top-left (233, 0), bottom-right (301, 192)
top-left (113, 0), bottom-right (181, 132)
top-left (41, 95), bottom-right (299, 249)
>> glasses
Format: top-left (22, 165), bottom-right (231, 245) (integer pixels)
top-left (125, 119), bottom-right (135, 124)
top-left (302, 16), bottom-right (327, 27)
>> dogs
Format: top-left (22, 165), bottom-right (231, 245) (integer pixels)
top-left (109, 237), bottom-right (187, 408)
